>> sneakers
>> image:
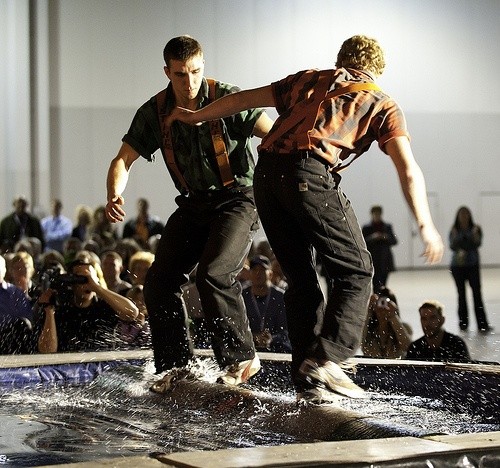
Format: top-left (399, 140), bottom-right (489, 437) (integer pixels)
top-left (299, 356), bottom-right (366, 399)
top-left (296, 386), bottom-right (350, 407)
top-left (217, 352), bottom-right (262, 386)
top-left (150, 361), bottom-right (203, 395)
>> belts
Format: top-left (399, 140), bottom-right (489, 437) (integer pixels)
top-left (184, 190), bottom-right (225, 202)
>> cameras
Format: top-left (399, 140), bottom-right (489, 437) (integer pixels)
top-left (374, 297), bottom-right (390, 310)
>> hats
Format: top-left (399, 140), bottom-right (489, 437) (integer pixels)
top-left (248, 255), bottom-right (271, 272)
